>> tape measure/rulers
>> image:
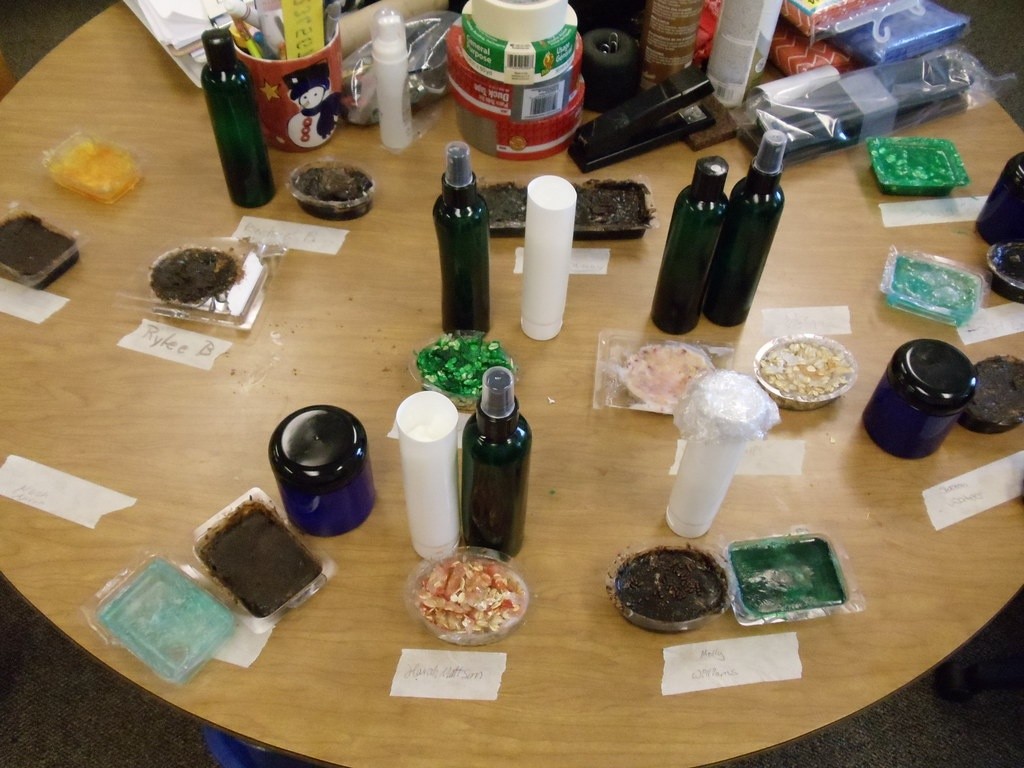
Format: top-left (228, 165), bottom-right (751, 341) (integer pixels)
top-left (280, 0), bottom-right (325, 60)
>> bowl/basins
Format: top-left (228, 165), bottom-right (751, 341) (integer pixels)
top-left (285, 158), bottom-right (379, 219)
top-left (987, 242), bottom-right (1024, 304)
top-left (607, 542), bottom-right (730, 635)
top-left (409, 551), bottom-right (532, 645)
top-left (752, 334), bottom-right (857, 410)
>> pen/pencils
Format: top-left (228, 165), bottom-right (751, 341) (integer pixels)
top-left (222, 0), bottom-right (346, 60)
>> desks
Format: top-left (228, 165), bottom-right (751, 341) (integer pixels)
top-left (0, 0), bottom-right (1024, 768)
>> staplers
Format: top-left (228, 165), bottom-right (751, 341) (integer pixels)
top-left (566, 62), bottom-right (716, 174)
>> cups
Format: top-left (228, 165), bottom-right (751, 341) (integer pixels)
top-left (230, 13), bottom-right (341, 152)
top-left (863, 338), bottom-right (976, 461)
top-left (265, 403), bottom-right (377, 533)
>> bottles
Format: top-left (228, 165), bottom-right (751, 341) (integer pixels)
top-left (464, 367), bottom-right (530, 556)
top-left (706, 131), bottom-right (787, 326)
top-left (653, 157), bottom-right (727, 334)
top-left (979, 152), bottom-right (1024, 241)
top-left (432, 142), bottom-right (489, 334)
top-left (200, 31), bottom-right (275, 205)
